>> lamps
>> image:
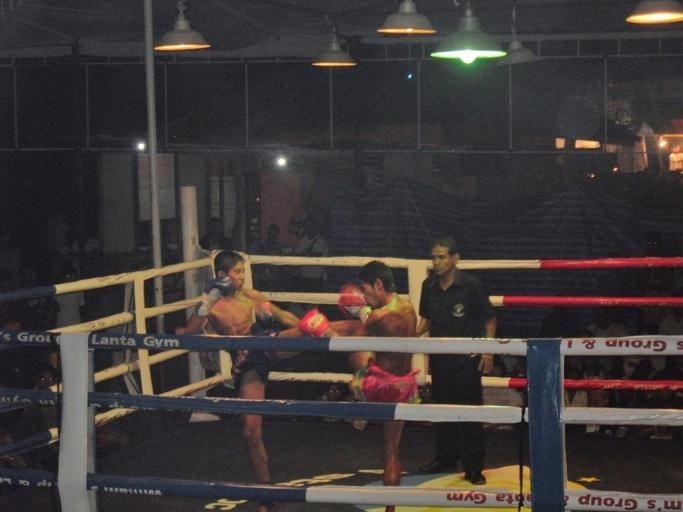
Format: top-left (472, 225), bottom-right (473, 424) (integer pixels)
top-left (622, 2), bottom-right (681, 26)
top-left (153, 0), bottom-right (211, 53)
top-left (311, 2), bottom-right (540, 71)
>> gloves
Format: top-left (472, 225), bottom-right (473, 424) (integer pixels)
top-left (338, 283), bottom-right (373, 324)
top-left (299, 310), bottom-right (332, 338)
top-left (193, 275), bottom-right (236, 318)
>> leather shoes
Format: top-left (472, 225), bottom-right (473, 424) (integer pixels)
top-left (420, 459), bottom-right (455, 474)
top-left (465, 471), bottom-right (486, 484)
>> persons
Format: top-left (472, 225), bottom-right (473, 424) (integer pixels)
top-left (297, 260), bottom-right (418, 512)
top-left (182, 250), bottom-right (365, 512)
top-left (197, 206), bottom-right (682, 443)
top-left (413, 236), bottom-right (498, 485)
top-left (0, 216), bottom-right (180, 499)
